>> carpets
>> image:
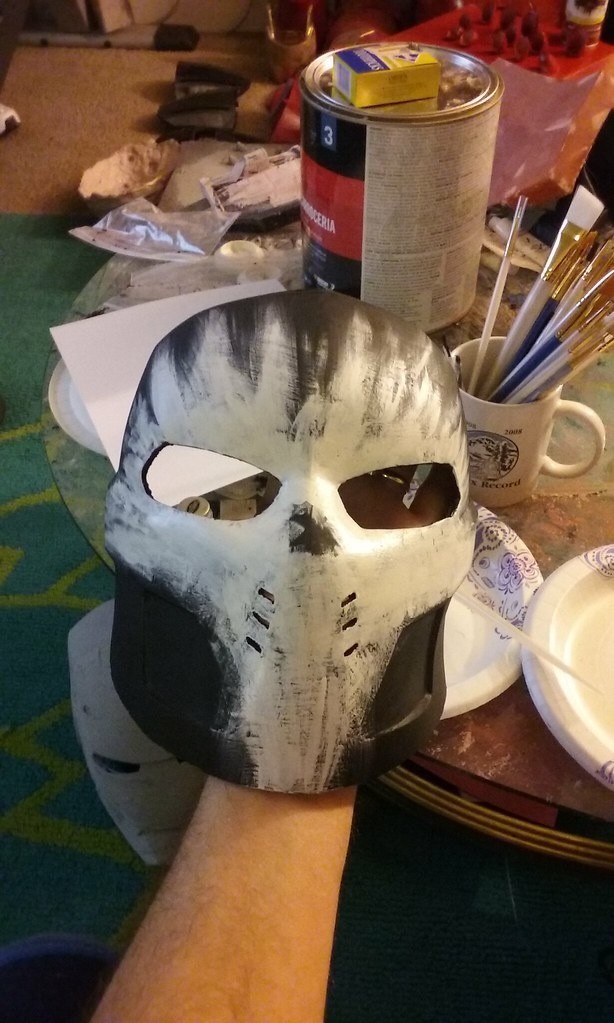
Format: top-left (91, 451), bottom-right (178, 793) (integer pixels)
top-left (0, 41), bottom-right (270, 218)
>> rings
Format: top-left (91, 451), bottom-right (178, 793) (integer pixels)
top-left (372, 470), bottom-right (410, 489)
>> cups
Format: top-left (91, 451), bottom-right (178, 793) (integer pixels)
top-left (448, 330), bottom-right (606, 510)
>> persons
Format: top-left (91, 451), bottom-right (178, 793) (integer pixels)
top-left (84, 461), bottom-right (456, 1023)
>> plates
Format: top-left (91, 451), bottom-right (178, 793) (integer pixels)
top-left (438, 498), bottom-right (544, 721)
top-left (517, 541), bottom-right (614, 796)
top-left (49, 356), bottom-right (110, 458)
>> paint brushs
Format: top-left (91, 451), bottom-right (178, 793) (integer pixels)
top-left (474, 193), bottom-right (614, 407)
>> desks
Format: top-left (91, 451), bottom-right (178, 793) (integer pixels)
top-left (38, 196), bottom-right (614, 866)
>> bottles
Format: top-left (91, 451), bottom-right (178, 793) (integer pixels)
top-left (564, 0), bottom-right (608, 49)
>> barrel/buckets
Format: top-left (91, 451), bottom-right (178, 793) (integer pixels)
top-left (297, 42), bottom-right (505, 338)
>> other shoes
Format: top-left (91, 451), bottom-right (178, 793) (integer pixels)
top-left (0, 103), bottom-right (23, 138)
